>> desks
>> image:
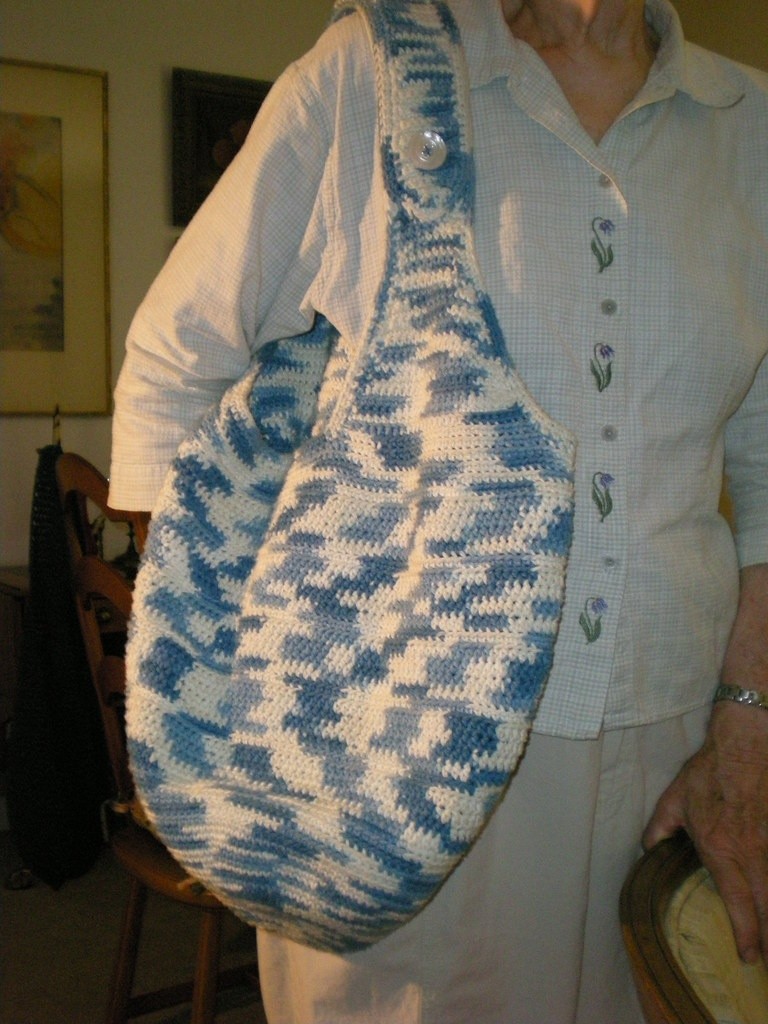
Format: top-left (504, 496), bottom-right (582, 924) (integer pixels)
top-left (0, 564), bottom-right (125, 722)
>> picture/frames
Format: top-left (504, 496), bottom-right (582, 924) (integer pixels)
top-left (0, 57), bottom-right (112, 419)
top-left (171, 67), bottom-right (273, 228)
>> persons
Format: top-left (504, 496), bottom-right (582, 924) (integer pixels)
top-left (111, 0), bottom-right (768, 1024)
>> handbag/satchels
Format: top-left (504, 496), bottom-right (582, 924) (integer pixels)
top-left (123, 7), bottom-right (581, 963)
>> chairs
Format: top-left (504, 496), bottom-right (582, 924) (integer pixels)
top-left (617, 835), bottom-right (768, 1023)
top-left (52, 453), bottom-right (224, 1024)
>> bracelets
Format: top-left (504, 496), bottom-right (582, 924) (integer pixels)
top-left (712, 684), bottom-right (768, 709)
